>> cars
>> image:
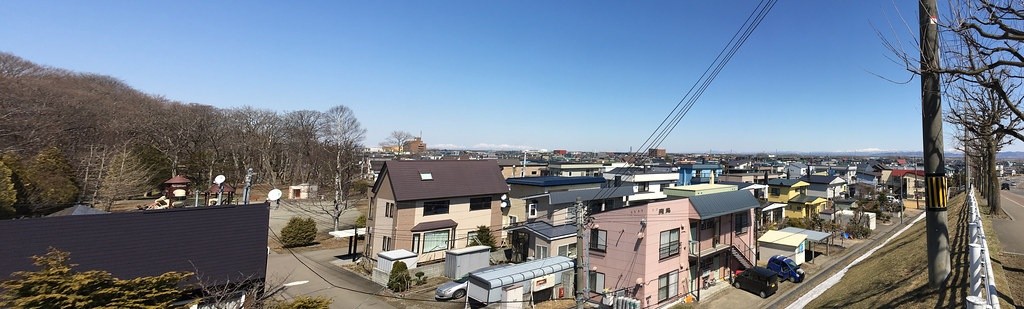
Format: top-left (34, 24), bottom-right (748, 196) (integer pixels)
top-left (886, 196), bottom-right (900, 204)
top-left (1000, 182), bottom-right (1010, 191)
top-left (434, 275), bottom-right (469, 301)
top-left (1006, 178), bottom-right (1017, 186)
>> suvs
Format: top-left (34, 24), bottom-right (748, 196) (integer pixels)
top-left (766, 254), bottom-right (806, 284)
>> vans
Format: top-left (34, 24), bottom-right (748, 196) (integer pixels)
top-left (731, 266), bottom-right (779, 298)
